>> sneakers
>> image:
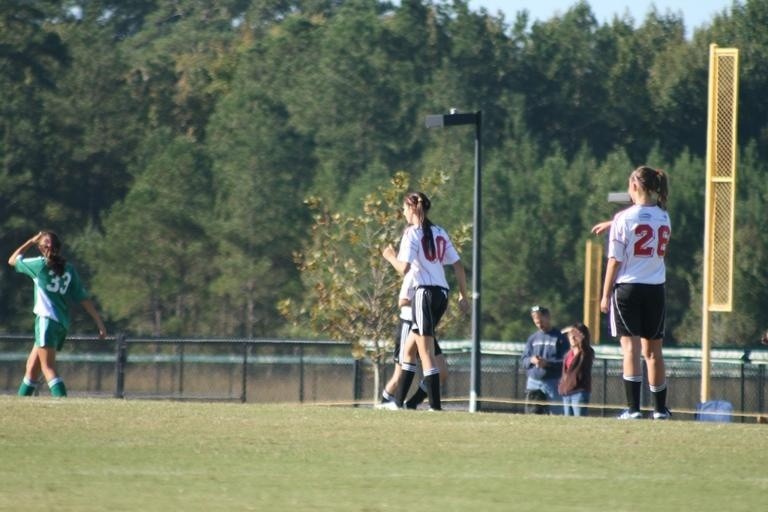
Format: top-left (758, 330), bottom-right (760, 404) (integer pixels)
top-left (616, 409), bottom-right (669, 419)
top-left (374, 401), bottom-right (404, 410)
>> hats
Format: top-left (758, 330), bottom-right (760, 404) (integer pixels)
top-left (529, 305), bottom-right (549, 314)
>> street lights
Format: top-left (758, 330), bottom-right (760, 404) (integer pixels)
top-left (426, 109), bottom-right (482, 413)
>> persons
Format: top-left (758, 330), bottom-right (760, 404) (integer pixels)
top-left (8, 232), bottom-right (106, 397)
top-left (380, 192), bottom-right (470, 411)
top-left (521, 305), bottom-right (570, 414)
top-left (592, 166), bottom-right (671, 419)
top-left (557, 323), bottom-right (594, 416)
